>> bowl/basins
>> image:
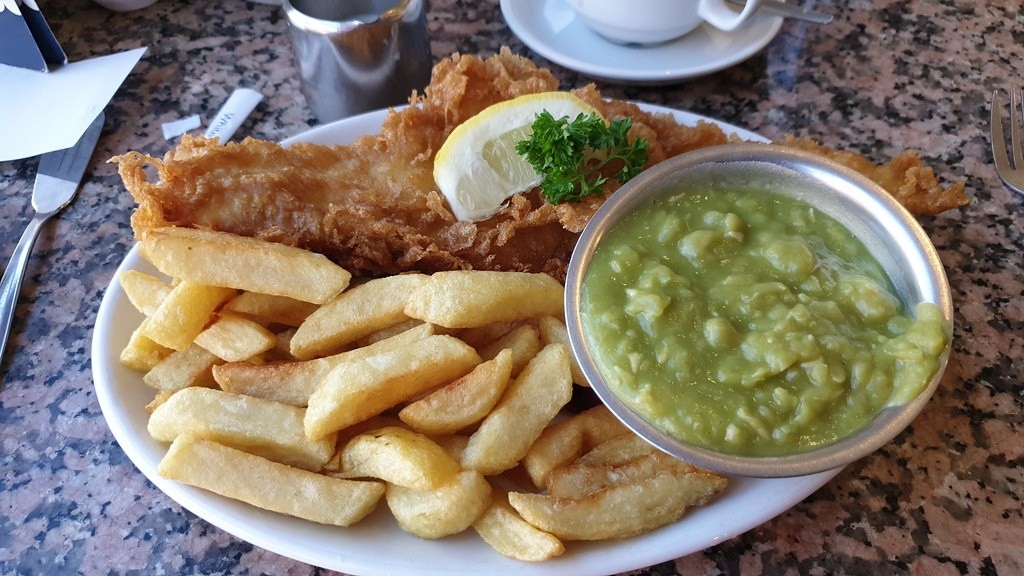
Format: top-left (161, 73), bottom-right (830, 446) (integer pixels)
top-left (564, 145), bottom-right (954, 479)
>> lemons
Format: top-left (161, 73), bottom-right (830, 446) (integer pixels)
top-left (432, 92), bottom-right (612, 224)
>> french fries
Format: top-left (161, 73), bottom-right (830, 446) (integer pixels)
top-left (119, 225), bottom-right (728, 562)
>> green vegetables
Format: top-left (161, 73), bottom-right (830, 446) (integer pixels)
top-left (514, 109), bottom-right (649, 206)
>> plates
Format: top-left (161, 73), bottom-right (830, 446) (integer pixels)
top-left (92, 99), bottom-right (847, 576)
top-left (499, 1), bottom-right (787, 81)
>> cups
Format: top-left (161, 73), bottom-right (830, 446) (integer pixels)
top-left (282, 1), bottom-right (431, 125)
top-left (571, 2), bottom-right (764, 45)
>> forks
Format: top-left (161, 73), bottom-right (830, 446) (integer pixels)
top-left (990, 87), bottom-right (1024, 197)
